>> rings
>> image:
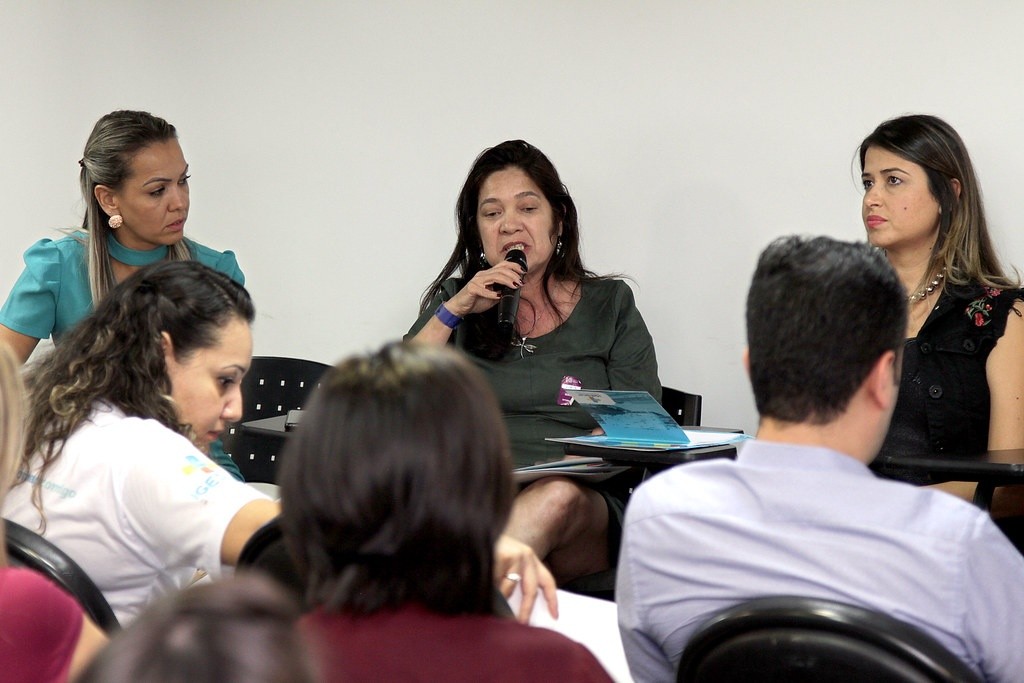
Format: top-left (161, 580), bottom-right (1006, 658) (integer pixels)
top-left (505, 571), bottom-right (524, 583)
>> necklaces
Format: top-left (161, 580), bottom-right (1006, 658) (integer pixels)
top-left (512, 281), bottom-right (558, 361)
top-left (908, 265), bottom-right (946, 306)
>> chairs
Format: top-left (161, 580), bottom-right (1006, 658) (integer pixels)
top-left (676, 587), bottom-right (993, 683)
top-left (224, 354), bottom-right (337, 480)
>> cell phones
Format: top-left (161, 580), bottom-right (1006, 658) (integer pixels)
top-left (285, 409), bottom-right (308, 431)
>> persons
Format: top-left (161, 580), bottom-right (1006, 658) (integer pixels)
top-left (0, 339), bottom-right (113, 683)
top-left (0, 259), bottom-right (561, 633)
top-left (399, 139), bottom-right (664, 552)
top-left (77, 575), bottom-right (316, 683)
top-left (618, 234), bottom-right (1024, 682)
top-left (0, 111), bottom-right (245, 483)
top-left (855, 113), bottom-right (1024, 552)
top-left (269, 338), bottom-right (615, 683)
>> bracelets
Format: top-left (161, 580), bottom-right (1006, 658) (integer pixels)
top-left (434, 303), bottom-right (463, 330)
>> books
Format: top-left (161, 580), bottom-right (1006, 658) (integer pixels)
top-left (511, 457), bottom-right (631, 485)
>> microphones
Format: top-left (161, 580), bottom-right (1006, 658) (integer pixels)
top-left (498, 249), bottom-right (528, 333)
